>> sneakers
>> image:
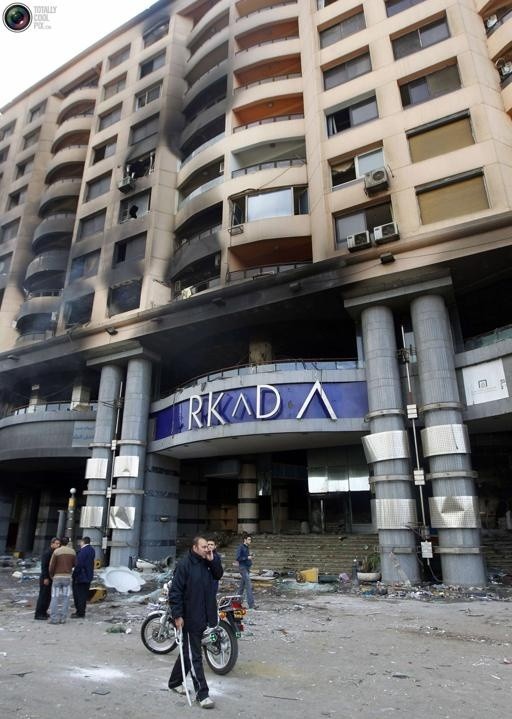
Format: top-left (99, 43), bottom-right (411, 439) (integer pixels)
top-left (173, 684), bottom-right (190, 695)
top-left (200, 698), bottom-right (214, 709)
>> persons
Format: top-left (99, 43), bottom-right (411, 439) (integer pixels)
top-left (236, 534), bottom-right (260, 610)
top-left (166, 535), bottom-right (223, 709)
top-left (70, 536), bottom-right (96, 618)
top-left (205, 536), bottom-right (223, 599)
top-left (47, 537), bottom-right (78, 625)
top-left (32, 537), bottom-right (62, 621)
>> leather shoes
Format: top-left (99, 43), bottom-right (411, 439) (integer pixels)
top-left (70, 614), bottom-right (81, 619)
top-left (35, 615), bottom-right (48, 620)
top-left (252, 605), bottom-right (259, 609)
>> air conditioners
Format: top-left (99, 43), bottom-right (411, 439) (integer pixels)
top-left (363, 167), bottom-right (390, 193)
top-left (117, 176), bottom-right (135, 193)
top-left (346, 221), bottom-right (400, 253)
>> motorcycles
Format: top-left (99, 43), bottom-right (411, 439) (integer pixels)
top-left (141, 571), bottom-right (248, 675)
